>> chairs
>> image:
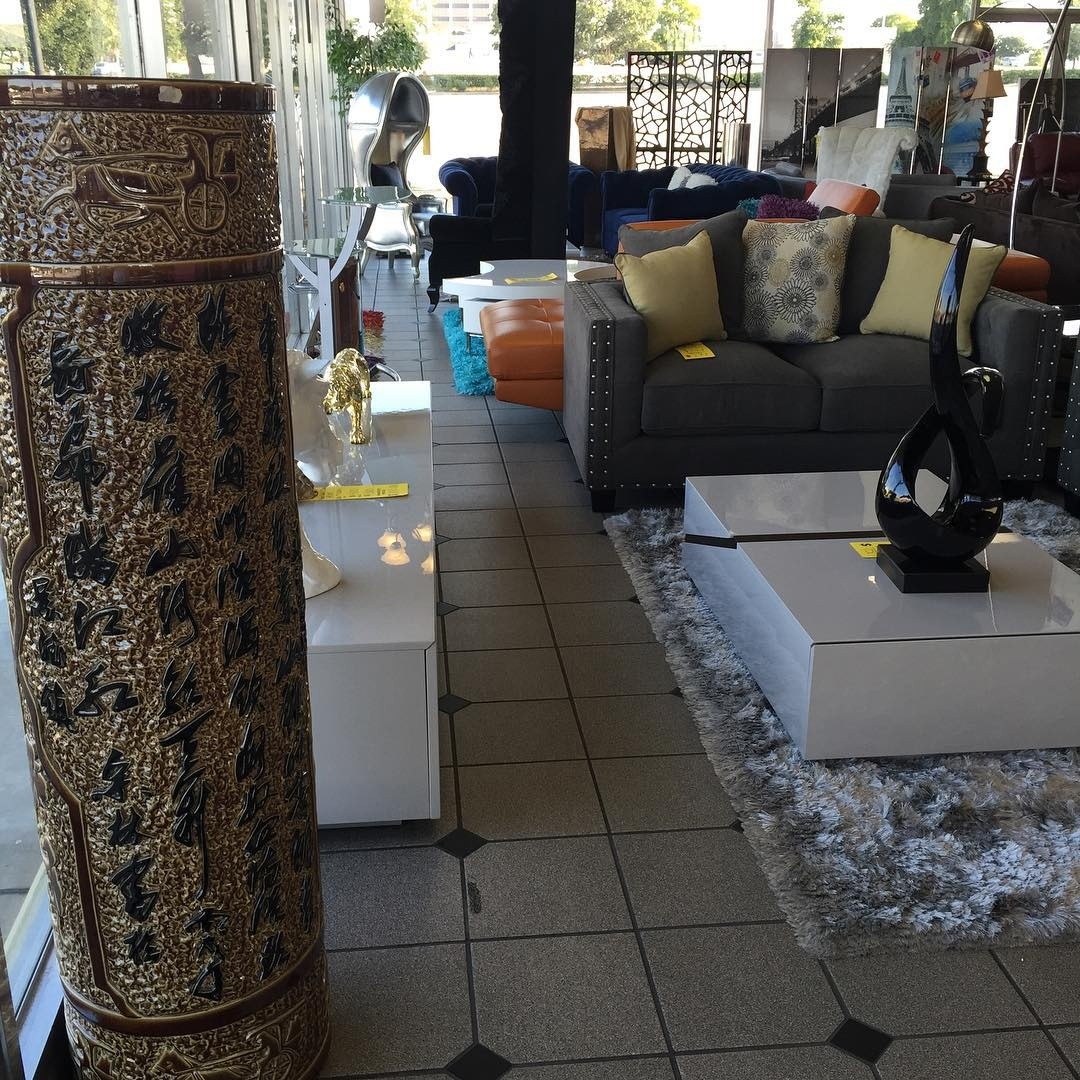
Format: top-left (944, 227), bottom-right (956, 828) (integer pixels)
top-left (350, 73), bottom-right (446, 284)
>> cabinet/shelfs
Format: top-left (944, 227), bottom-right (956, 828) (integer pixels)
top-left (277, 183), bottom-right (411, 381)
top-left (296, 378), bottom-right (442, 828)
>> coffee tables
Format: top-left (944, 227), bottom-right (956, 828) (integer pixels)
top-left (443, 260), bottom-right (612, 354)
top-left (683, 468), bottom-right (1080, 760)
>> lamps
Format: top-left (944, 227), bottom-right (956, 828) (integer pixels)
top-left (949, 0), bottom-right (1067, 196)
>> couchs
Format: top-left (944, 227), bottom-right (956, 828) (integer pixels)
top-left (430, 126), bottom-right (1080, 512)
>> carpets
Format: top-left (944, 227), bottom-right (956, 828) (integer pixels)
top-left (439, 306), bottom-right (493, 395)
top-left (603, 493), bottom-right (1079, 955)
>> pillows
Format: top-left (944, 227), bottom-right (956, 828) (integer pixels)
top-left (666, 166), bottom-right (691, 191)
top-left (961, 169), bottom-right (1021, 204)
top-left (1032, 178), bottom-right (1080, 224)
top-left (614, 229), bottom-right (729, 363)
top-left (740, 215), bottom-right (859, 343)
top-left (977, 176), bottom-right (1037, 214)
top-left (687, 174), bottom-right (717, 190)
top-left (860, 224), bottom-right (1006, 357)
top-left (616, 207), bottom-right (748, 339)
top-left (370, 159), bottom-right (404, 192)
